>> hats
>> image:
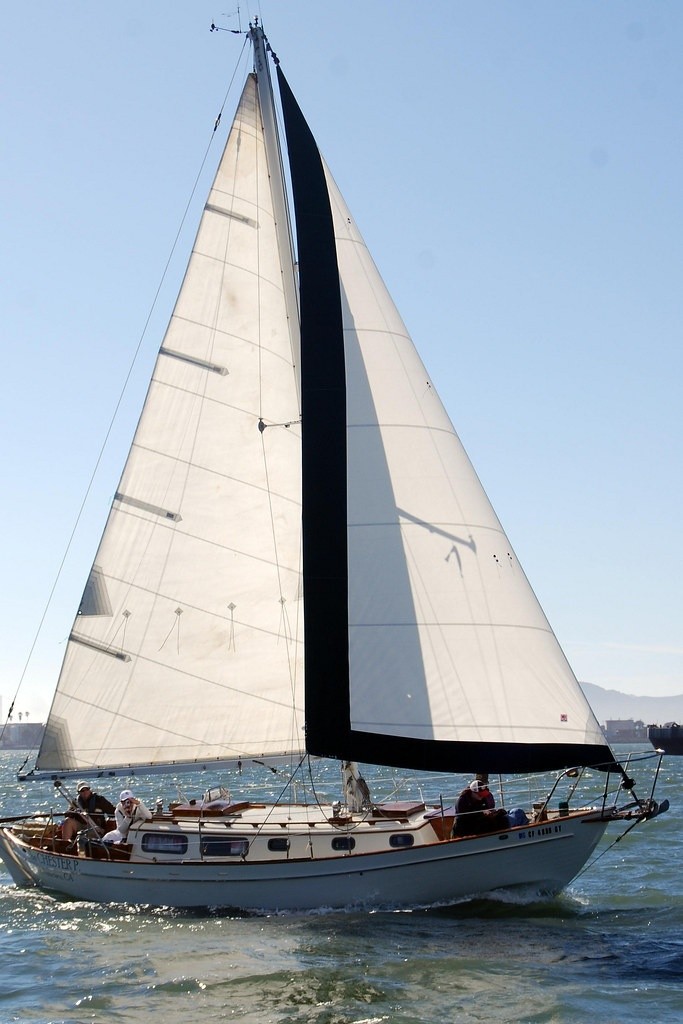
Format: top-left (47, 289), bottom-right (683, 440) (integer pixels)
top-left (475, 773), bottom-right (489, 784)
top-left (76, 780), bottom-right (90, 791)
top-left (120, 790), bottom-right (134, 802)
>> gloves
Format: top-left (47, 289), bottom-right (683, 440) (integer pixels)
top-left (481, 810), bottom-right (490, 815)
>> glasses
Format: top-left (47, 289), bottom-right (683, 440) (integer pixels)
top-left (79, 787), bottom-right (91, 793)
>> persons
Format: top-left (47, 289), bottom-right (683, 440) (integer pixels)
top-left (101, 790), bottom-right (149, 848)
top-left (60, 781), bottom-right (119, 851)
top-left (452, 772), bottom-right (530, 841)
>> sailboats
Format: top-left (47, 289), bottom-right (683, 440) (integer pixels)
top-left (1, 4), bottom-right (671, 914)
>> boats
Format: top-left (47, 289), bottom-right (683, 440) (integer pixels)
top-left (648, 723), bottom-right (683, 756)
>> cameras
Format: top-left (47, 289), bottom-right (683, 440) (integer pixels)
top-left (125, 800), bottom-right (130, 806)
